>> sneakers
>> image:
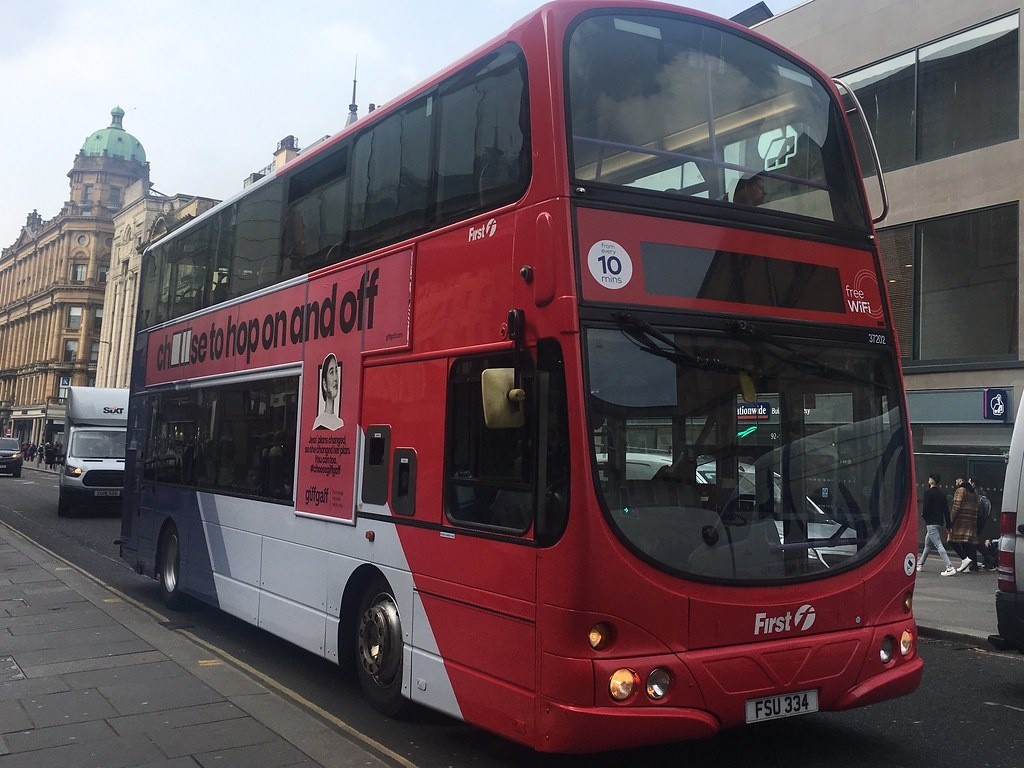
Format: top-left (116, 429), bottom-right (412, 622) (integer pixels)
top-left (962, 566), bottom-right (970, 573)
top-left (941, 567), bottom-right (956, 576)
top-left (916, 564), bottom-right (923, 572)
top-left (957, 557), bottom-right (972, 572)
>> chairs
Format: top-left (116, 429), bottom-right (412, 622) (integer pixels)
top-left (213, 272), bottom-right (244, 304)
top-left (324, 239), bottom-right (362, 261)
top-left (192, 281), bottom-right (225, 311)
top-left (173, 288), bottom-right (202, 318)
top-left (479, 160), bottom-right (519, 207)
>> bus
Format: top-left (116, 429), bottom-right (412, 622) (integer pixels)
top-left (108, 0), bottom-right (926, 757)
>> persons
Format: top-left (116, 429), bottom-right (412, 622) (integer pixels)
top-left (322, 353), bottom-right (339, 415)
top-left (915, 473), bottom-right (956, 577)
top-left (21, 441), bottom-right (63, 464)
top-left (733, 174), bottom-right (767, 206)
top-left (947, 474), bottom-right (1001, 573)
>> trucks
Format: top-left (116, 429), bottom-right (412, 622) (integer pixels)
top-left (45, 385), bottom-right (130, 518)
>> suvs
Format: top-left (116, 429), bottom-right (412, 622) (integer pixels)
top-left (0, 437), bottom-right (26, 479)
top-left (591, 451), bottom-right (879, 571)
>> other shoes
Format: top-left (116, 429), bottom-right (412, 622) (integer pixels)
top-left (971, 564), bottom-right (979, 572)
top-left (987, 565), bottom-right (997, 571)
